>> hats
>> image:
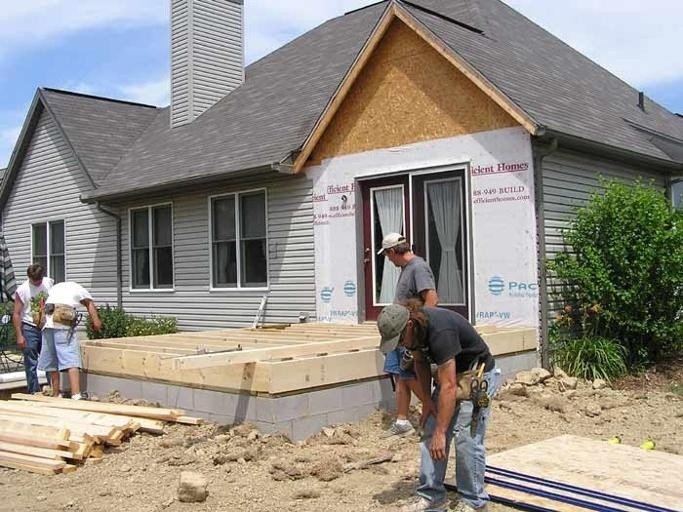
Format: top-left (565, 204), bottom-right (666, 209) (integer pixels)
top-left (376, 233), bottom-right (407, 256)
top-left (377, 304), bottom-right (410, 354)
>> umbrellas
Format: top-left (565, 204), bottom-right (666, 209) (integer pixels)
top-left (0, 233), bottom-right (18, 304)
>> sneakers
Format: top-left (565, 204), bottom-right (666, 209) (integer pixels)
top-left (396, 495), bottom-right (488, 512)
top-left (376, 418), bottom-right (416, 442)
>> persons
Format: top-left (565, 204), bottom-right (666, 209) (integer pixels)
top-left (11, 264), bottom-right (55, 394)
top-left (376, 298), bottom-right (497, 511)
top-left (375, 233), bottom-right (440, 440)
top-left (36, 280), bottom-right (101, 400)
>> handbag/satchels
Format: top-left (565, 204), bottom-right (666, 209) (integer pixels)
top-left (53, 304), bottom-right (76, 326)
top-left (455, 370), bottom-right (484, 402)
